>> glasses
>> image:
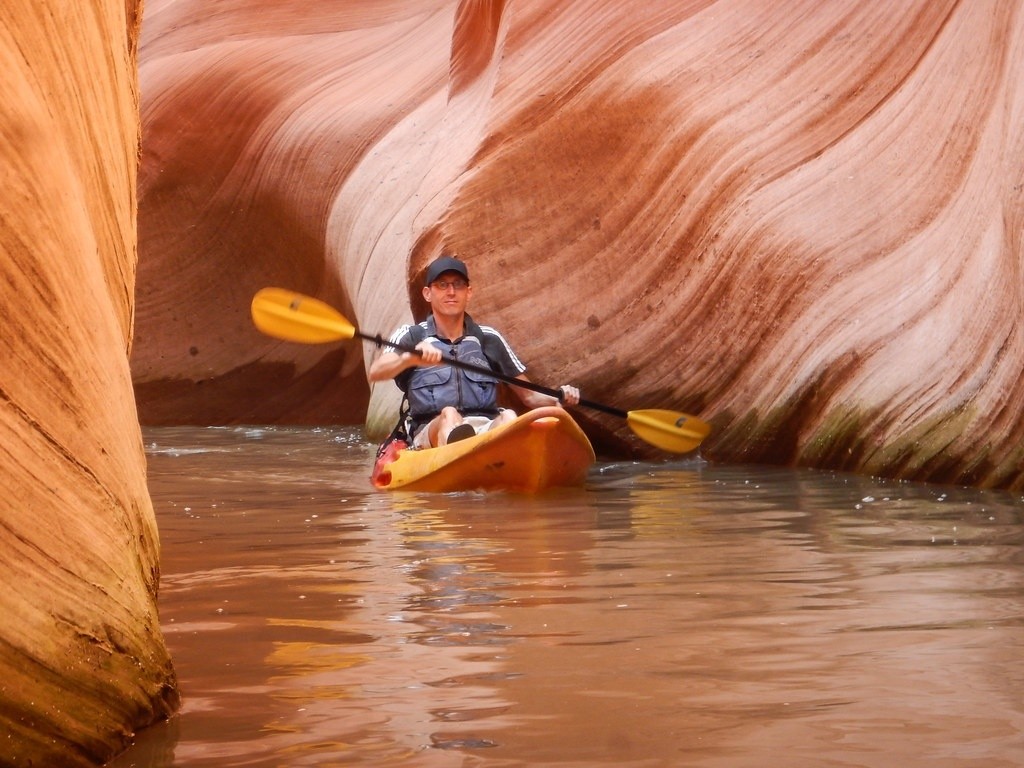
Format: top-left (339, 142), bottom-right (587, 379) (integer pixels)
top-left (431, 279), bottom-right (467, 290)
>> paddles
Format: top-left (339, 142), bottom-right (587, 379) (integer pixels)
top-left (251, 285), bottom-right (714, 458)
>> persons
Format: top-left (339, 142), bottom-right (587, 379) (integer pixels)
top-left (369, 257), bottom-right (580, 451)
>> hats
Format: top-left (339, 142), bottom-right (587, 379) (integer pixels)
top-left (428, 257), bottom-right (468, 287)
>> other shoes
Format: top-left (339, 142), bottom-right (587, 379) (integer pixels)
top-left (446, 424), bottom-right (476, 444)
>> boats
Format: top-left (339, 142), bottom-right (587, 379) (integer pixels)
top-left (369, 406), bottom-right (596, 494)
